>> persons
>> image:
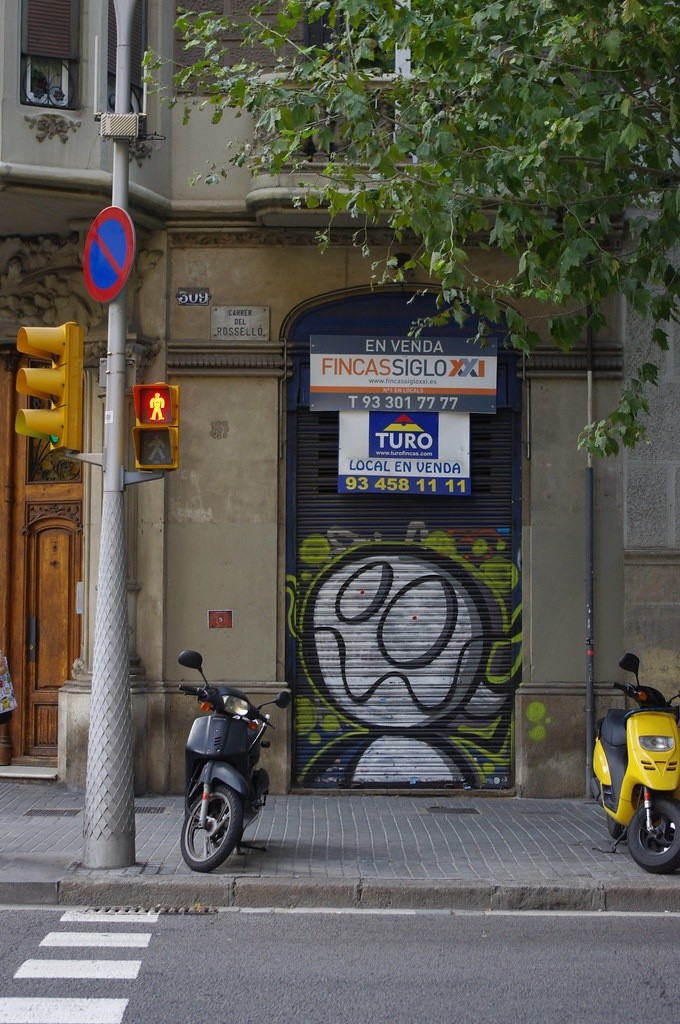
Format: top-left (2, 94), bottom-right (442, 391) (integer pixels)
top-left (0, 647), bottom-right (14, 726)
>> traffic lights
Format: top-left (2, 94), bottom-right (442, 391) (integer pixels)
top-left (131, 378), bottom-right (180, 471)
top-left (14, 321), bottom-right (87, 453)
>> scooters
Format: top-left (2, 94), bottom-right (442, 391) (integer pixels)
top-left (593, 651), bottom-right (680, 873)
top-left (177, 649), bottom-right (291, 872)
